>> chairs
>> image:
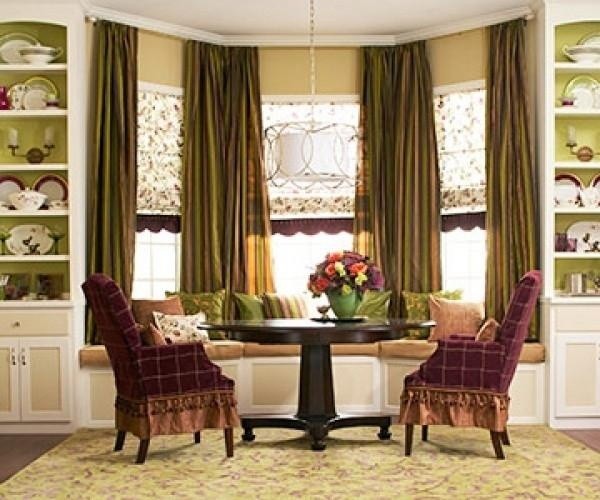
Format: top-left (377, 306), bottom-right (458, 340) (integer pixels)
top-left (81, 272), bottom-right (243, 466)
top-left (398, 270), bottom-right (543, 461)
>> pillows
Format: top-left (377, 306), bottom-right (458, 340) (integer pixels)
top-left (131, 287), bottom-right (227, 347)
top-left (231, 291), bottom-right (310, 320)
top-left (355, 288), bottom-right (393, 318)
top-left (401, 290), bottom-right (499, 342)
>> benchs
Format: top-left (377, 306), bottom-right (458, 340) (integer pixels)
top-left (242, 339), bottom-right (383, 419)
top-left (78, 338), bottom-right (242, 430)
top-left (378, 339), bottom-right (547, 425)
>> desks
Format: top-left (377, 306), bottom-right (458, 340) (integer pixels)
top-left (197, 317), bottom-right (438, 451)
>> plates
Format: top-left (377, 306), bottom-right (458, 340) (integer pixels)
top-left (554, 29), bottom-right (600, 257)
top-left (0, 32), bottom-right (67, 255)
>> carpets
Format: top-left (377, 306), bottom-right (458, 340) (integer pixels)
top-left (0, 424), bottom-right (599, 500)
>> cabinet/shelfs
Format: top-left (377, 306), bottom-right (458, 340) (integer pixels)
top-left (531, 0), bottom-right (600, 432)
top-left (0, 1), bottom-right (81, 434)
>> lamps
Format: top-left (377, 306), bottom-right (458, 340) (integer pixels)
top-left (258, 0), bottom-right (364, 190)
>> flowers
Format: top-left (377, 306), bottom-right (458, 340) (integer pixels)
top-left (307, 251), bottom-right (385, 296)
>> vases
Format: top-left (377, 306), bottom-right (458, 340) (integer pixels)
top-left (323, 290), bottom-right (363, 319)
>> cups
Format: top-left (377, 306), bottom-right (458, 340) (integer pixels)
top-left (1, 272), bottom-right (65, 301)
top-left (563, 272), bottom-right (588, 294)
